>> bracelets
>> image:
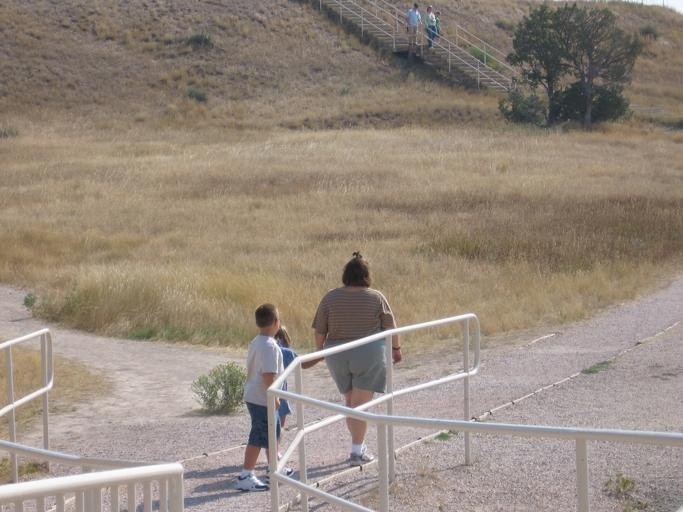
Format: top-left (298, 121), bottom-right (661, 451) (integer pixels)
top-left (392, 344), bottom-right (401, 351)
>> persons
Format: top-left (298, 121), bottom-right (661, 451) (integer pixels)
top-left (405, 4), bottom-right (422, 45)
top-left (434, 12), bottom-right (440, 34)
top-left (424, 6), bottom-right (437, 48)
top-left (311, 249), bottom-right (401, 466)
top-left (233, 303), bottom-right (294, 492)
top-left (273, 323), bottom-right (324, 462)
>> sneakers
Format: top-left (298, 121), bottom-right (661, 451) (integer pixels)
top-left (349, 450), bottom-right (374, 467)
top-left (266, 467), bottom-right (294, 485)
top-left (234, 472), bottom-right (270, 491)
